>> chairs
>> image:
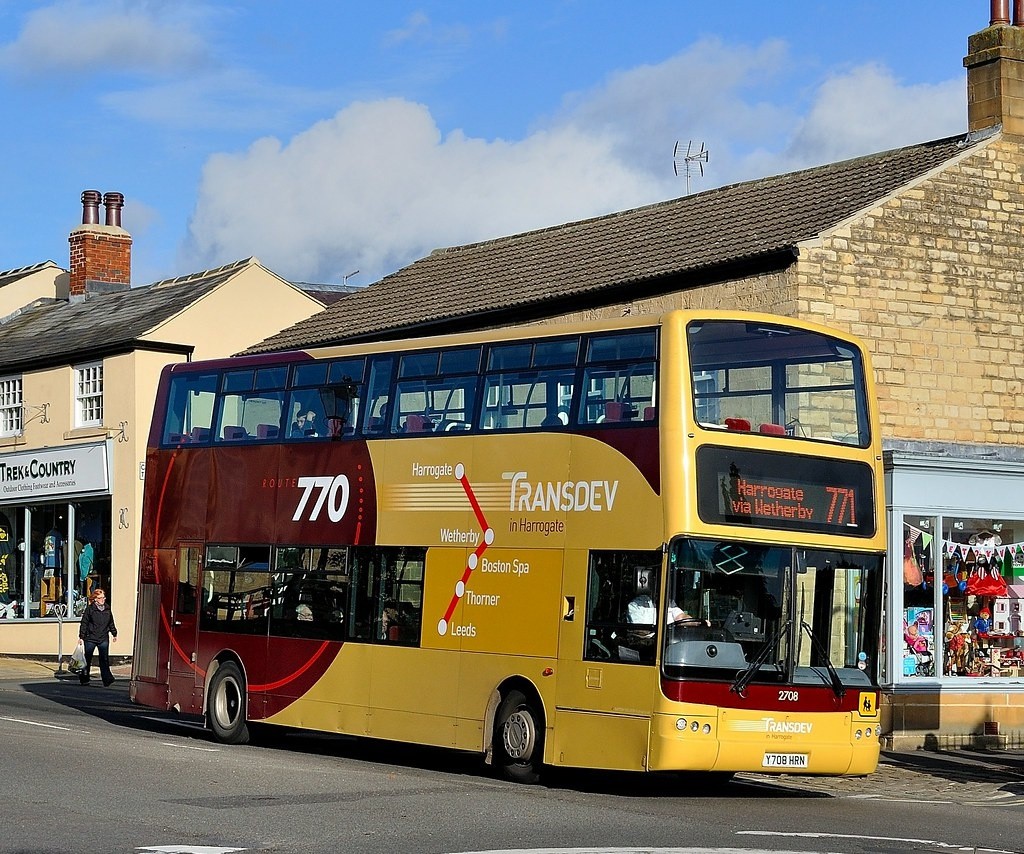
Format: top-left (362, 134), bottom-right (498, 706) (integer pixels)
top-left (168, 402), bottom-right (656, 443)
top-left (725, 418), bottom-right (751, 432)
top-left (759, 423), bottom-right (786, 436)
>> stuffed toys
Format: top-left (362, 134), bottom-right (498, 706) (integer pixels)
top-left (973, 607), bottom-right (992, 635)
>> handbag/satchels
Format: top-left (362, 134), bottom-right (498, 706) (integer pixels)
top-left (68, 640), bottom-right (88, 676)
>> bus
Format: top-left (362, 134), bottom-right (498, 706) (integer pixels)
top-left (129, 310), bottom-right (887, 785)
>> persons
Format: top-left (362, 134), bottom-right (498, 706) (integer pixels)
top-left (296, 604), bottom-right (313, 621)
top-left (357, 610), bottom-right (388, 639)
top-left (290, 409), bottom-right (318, 437)
top-left (626, 571), bottom-right (711, 645)
top-left (78, 589), bottom-right (117, 687)
top-left (908, 626), bottom-right (924, 646)
top-left (377, 403), bottom-right (387, 433)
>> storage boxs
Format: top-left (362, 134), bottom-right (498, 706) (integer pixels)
top-left (907, 607), bottom-right (934, 635)
